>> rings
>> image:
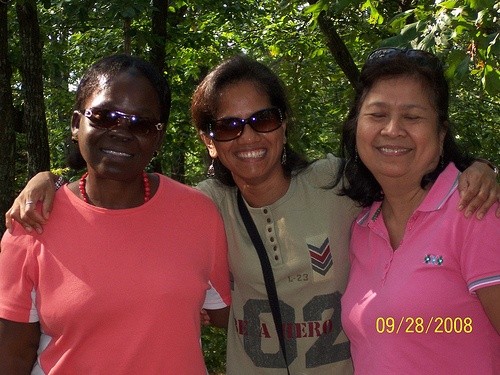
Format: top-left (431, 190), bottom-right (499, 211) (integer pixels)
top-left (25, 201), bottom-right (32, 205)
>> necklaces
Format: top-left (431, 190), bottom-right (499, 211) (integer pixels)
top-left (79, 169), bottom-right (150, 207)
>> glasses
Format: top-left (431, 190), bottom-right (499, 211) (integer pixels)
top-left (78, 107), bottom-right (167, 140)
top-left (206, 107), bottom-right (286, 142)
top-left (364, 47), bottom-right (440, 66)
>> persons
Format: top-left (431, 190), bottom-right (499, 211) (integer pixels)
top-left (0, 52), bottom-right (232, 375)
top-left (339, 48), bottom-right (500, 375)
top-left (5, 52), bottom-right (500, 375)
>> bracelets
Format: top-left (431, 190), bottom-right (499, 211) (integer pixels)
top-left (54, 177), bottom-right (65, 191)
